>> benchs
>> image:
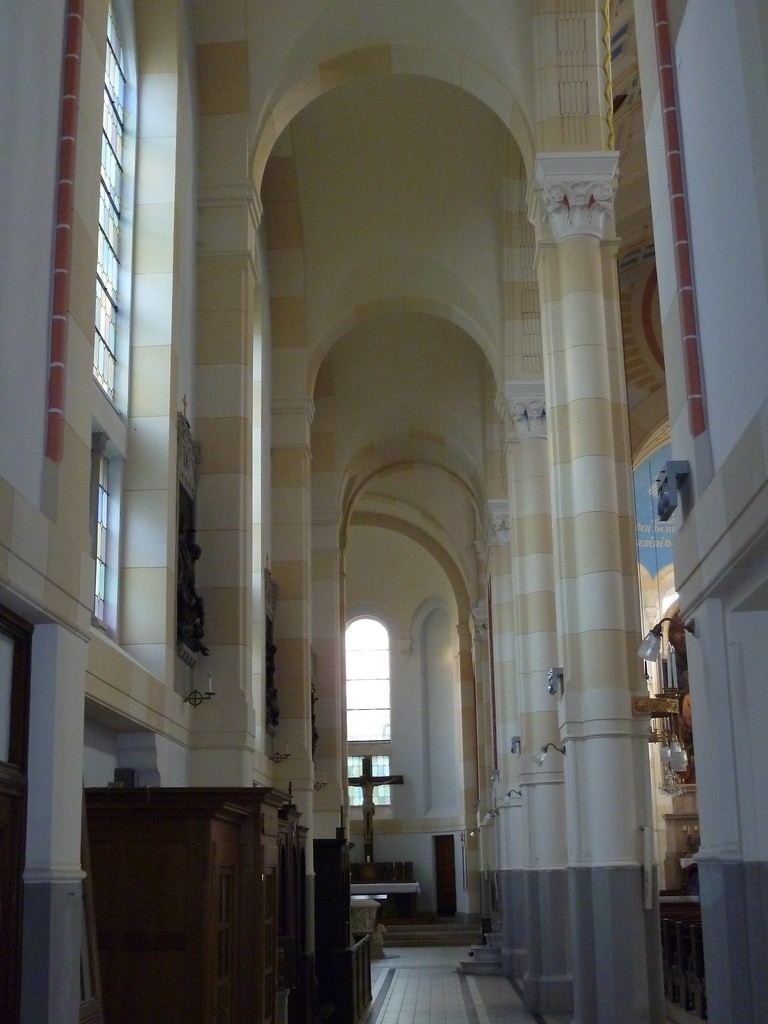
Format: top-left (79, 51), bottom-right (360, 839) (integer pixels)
top-left (660, 918), bottom-right (707, 1019)
top-left (351, 894), bottom-right (387, 922)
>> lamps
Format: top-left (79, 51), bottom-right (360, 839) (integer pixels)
top-left (490, 769), bottom-right (499, 782)
top-left (470, 828), bottom-right (480, 836)
top-left (487, 809), bottom-right (499, 819)
top-left (638, 618), bottom-right (695, 663)
top-left (532, 743), bottom-right (565, 766)
top-left (656, 460), bottom-right (690, 521)
top-left (504, 790), bottom-right (521, 803)
top-left (649, 688), bottom-right (688, 797)
top-left (547, 668), bottom-right (563, 695)
top-left (511, 736), bottom-right (520, 753)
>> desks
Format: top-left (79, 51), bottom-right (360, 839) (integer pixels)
top-left (350, 882), bottom-right (421, 918)
top-left (678, 858), bottom-right (700, 895)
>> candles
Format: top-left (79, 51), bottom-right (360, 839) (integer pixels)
top-left (208, 672), bottom-right (212, 692)
top-left (688, 826), bottom-right (690, 835)
top-left (285, 742), bottom-right (288, 754)
top-left (683, 826), bottom-right (686, 830)
top-left (324, 773), bottom-right (326, 783)
top-left (694, 826), bottom-right (697, 830)
